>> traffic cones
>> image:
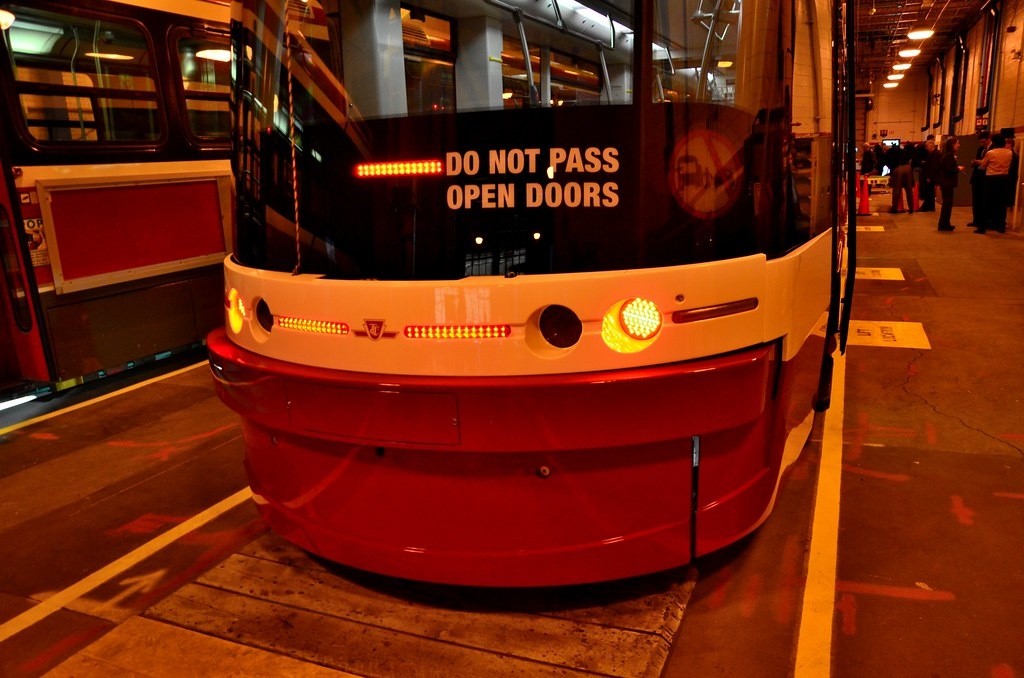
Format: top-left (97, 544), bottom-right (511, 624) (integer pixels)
top-left (855, 171), bottom-right (864, 200)
top-left (896, 189), bottom-right (906, 214)
top-left (912, 180), bottom-right (920, 213)
top-left (856, 174), bottom-right (874, 219)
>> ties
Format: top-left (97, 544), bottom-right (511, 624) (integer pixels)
top-left (982, 148), bottom-right (987, 159)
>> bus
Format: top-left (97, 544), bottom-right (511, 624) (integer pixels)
top-left (204, 2), bottom-right (873, 591)
top-left (0, 0), bottom-right (243, 400)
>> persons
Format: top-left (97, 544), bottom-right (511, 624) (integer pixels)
top-left (904, 140), bottom-right (915, 148)
top-left (973, 134), bottom-right (1013, 234)
top-left (860, 141), bottom-right (874, 196)
top-left (888, 149), bottom-right (914, 214)
top-left (919, 140), bottom-right (943, 211)
top-left (936, 137), bottom-right (966, 231)
top-left (986, 137), bottom-right (1019, 230)
top-left (967, 133), bottom-right (994, 226)
top-left (873, 144), bottom-right (883, 167)
top-left (884, 144), bottom-right (903, 187)
top-left (916, 134), bottom-right (935, 168)
top-left (882, 143), bottom-right (891, 154)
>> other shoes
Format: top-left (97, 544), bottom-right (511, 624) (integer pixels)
top-left (967, 222), bottom-right (974, 226)
top-left (974, 228), bottom-right (986, 233)
top-left (916, 207), bottom-right (935, 212)
top-left (938, 225), bottom-right (955, 230)
